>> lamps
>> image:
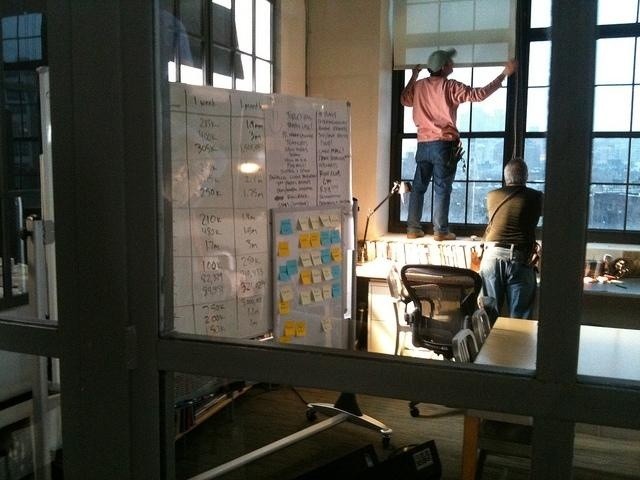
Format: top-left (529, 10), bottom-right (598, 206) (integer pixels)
top-left (360, 180), bottom-right (414, 263)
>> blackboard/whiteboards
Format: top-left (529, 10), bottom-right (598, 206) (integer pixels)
top-left (169, 82), bottom-right (354, 349)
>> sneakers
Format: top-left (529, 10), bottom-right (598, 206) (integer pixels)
top-left (408, 228), bottom-right (425, 240)
top-left (431, 231), bottom-right (457, 242)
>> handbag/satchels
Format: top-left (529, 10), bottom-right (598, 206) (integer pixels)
top-left (480, 221), bottom-right (493, 249)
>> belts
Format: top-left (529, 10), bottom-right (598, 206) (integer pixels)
top-left (484, 242), bottom-right (531, 251)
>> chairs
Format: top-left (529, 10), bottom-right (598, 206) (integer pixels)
top-left (400, 264), bottom-right (482, 416)
top-left (452, 308), bottom-right (535, 480)
top-left (387, 264), bottom-right (450, 356)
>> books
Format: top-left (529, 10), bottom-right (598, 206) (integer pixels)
top-left (363, 238), bottom-right (484, 273)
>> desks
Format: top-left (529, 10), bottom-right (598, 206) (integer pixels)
top-left (462, 316), bottom-right (640, 480)
top-left (355, 267), bottom-right (640, 362)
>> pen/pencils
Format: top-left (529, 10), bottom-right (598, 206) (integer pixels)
top-left (616, 284), bottom-right (627, 289)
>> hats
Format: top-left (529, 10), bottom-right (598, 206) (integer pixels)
top-left (426, 47), bottom-right (457, 73)
top-left (503, 156), bottom-right (529, 186)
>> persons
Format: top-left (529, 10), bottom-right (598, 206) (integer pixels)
top-left (400, 47), bottom-right (518, 241)
top-left (476, 158), bottom-right (544, 318)
